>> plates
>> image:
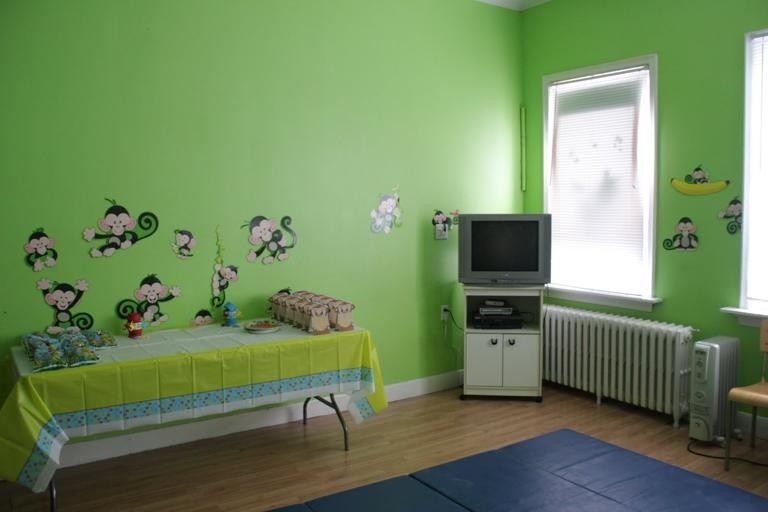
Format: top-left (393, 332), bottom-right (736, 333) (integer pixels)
top-left (244, 320), bottom-right (283, 333)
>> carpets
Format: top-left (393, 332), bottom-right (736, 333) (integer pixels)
top-left (410, 428), bottom-right (767, 511)
top-left (268, 474), bottom-right (470, 512)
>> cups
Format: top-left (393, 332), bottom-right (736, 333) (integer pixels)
top-left (128, 313), bottom-right (141, 338)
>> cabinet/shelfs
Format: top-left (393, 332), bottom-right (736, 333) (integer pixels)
top-left (460, 284), bottom-right (546, 402)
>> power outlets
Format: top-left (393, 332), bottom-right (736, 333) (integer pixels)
top-left (441, 305), bottom-right (448, 320)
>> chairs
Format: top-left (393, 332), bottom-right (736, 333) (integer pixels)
top-left (725, 319), bottom-right (768, 471)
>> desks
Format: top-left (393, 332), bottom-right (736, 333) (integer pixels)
top-left (0, 318), bottom-right (388, 512)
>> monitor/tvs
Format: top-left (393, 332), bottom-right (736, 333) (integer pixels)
top-left (458, 214), bottom-right (551, 285)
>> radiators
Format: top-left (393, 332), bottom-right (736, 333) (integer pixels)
top-left (543, 304), bottom-right (701, 428)
top-left (689, 336), bottom-right (743, 448)
